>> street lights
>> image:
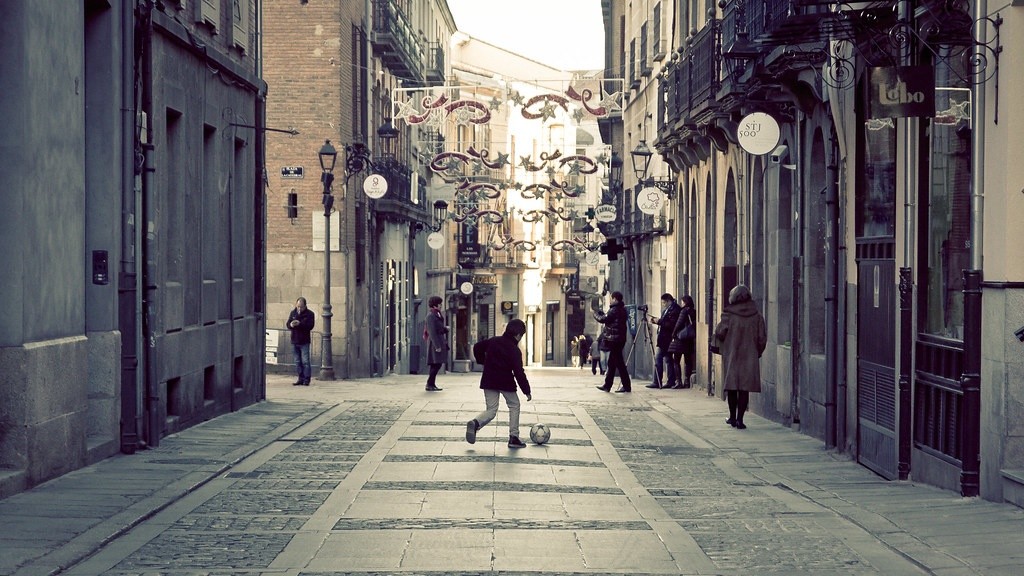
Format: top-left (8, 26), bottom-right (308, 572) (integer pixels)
top-left (316, 137), bottom-right (337, 382)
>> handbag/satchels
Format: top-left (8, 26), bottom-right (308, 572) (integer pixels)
top-left (709, 334), bottom-right (720, 354)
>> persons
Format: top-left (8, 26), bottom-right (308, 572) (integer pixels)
top-left (571, 334), bottom-right (593, 369)
top-left (714, 285), bottom-right (766, 428)
top-left (466, 319), bottom-right (531, 446)
top-left (590, 337), bottom-right (602, 375)
top-left (645, 293), bottom-right (680, 389)
top-left (425, 296), bottom-right (450, 391)
top-left (663, 295), bottom-right (696, 388)
top-left (589, 291), bottom-right (631, 393)
top-left (286, 297), bottom-right (314, 385)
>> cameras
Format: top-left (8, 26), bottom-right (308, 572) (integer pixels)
top-left (638, 304), bottom-right (648, 311)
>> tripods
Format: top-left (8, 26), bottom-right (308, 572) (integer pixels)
top-left (617, 311), bottom-right (662, 390)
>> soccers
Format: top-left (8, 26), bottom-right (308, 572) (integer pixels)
top-left (530, 423), bottom-right (551, 444)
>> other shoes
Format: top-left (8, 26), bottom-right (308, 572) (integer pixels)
top-left (596, 385), bottom-right (609, 392)
top-left (736, 421), bottom-right (746, 429)
top-left (303, 380), bottom-right (308, 386)
top-left (646, 382), bottom-right (660, 387)
top-left (293, 380), bottom-right (302, 385)
top-left (725, 416), bottom-right (736, 427)
top-left (661, 381), bottom-right (674, 388)
top-left (671, 380), bottom-right (682, 389)
top-left (466, 419), bottom-right (477, 443)
top-left (508, 437), bottom-right (526, 448)
top-left (615, 387), bottom-right (630, 393)
top-left (426, 384), bottom-right (442, 391)
top-left (684, 380), bottom-right (690, 389)
